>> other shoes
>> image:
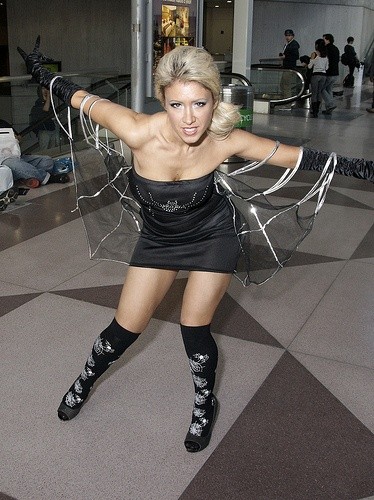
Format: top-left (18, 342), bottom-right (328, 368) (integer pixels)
top-left (184, 395), bottom-right (217, 452)
top-left (279, 103), bottom-right (295, 111)
top-left (57, 394), bottom-right (85, 421)
top-left (367, 107), bottom-right (374, 113)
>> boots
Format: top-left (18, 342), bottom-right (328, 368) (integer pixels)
top-left (310, 100), bottom-right (321, 118)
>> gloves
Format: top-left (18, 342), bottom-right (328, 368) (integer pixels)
top-left (298, 147), bottom-right (374, 183)
top-left (17, 34), bottom-right (86, 110)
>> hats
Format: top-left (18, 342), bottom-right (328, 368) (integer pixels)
top-left (285, 30), bottom-right (293, 34)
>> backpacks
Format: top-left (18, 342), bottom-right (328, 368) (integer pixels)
top-left (341, 45), bottom-right (352, 65)
top-left (29, 100), bottom-right (45, 134)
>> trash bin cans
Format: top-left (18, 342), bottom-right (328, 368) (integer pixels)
top-left (220, 83), bottom-right (254, 164)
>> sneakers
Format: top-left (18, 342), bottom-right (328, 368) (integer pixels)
top-left (48, 172), bottom-right (69, 184)
top-left (326, 105), bottom-right (337, 113)
top-left (0, 185), bottom-right (18, 211)
top-left (16, 179), bottom-right (39, 188)
top-left (322, 109), bottom-right (331, 114)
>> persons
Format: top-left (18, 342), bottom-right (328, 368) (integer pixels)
top-left (279, 30), bottom-right (300, 111)
top-left (308, 38), bottom-right (329, 118)
top-left (344, 36), bottom-right (360, 89)
top-left (16, 35), bottom-right (374, 452)
top-left (322, 33), bottom-right (340, 115)
top-left (35, 84), bottom-right (56, 152)
top-left (0, 119), bottom-right (69, 188)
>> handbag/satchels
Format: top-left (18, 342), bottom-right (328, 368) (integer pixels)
top-left (52, 157), bottom-right (75, 173)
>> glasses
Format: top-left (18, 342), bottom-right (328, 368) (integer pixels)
top-left (285, 35), bottom-right (292, 36)
